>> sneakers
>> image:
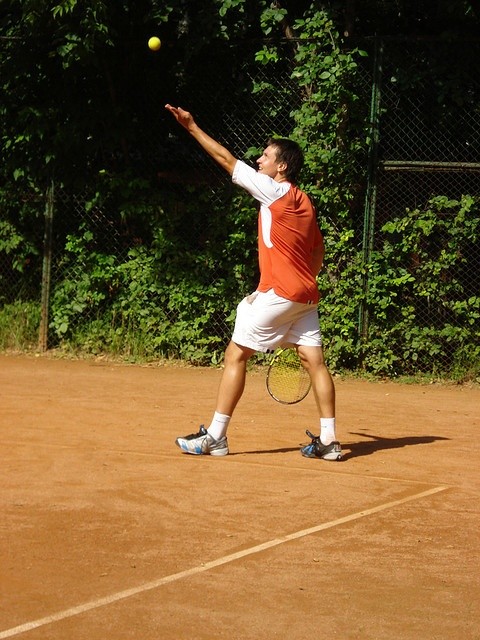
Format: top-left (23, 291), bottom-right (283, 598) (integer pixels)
top-left (175, 424), bottom-right (229, 456)
top-left (301, 429), bottom-right (344, 460)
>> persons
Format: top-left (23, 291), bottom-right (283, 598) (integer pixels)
top-left (165, 101), bottom-right (343, 461)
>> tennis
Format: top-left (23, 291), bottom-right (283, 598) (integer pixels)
top-left (148, 36), bottom-right (161, 51)
